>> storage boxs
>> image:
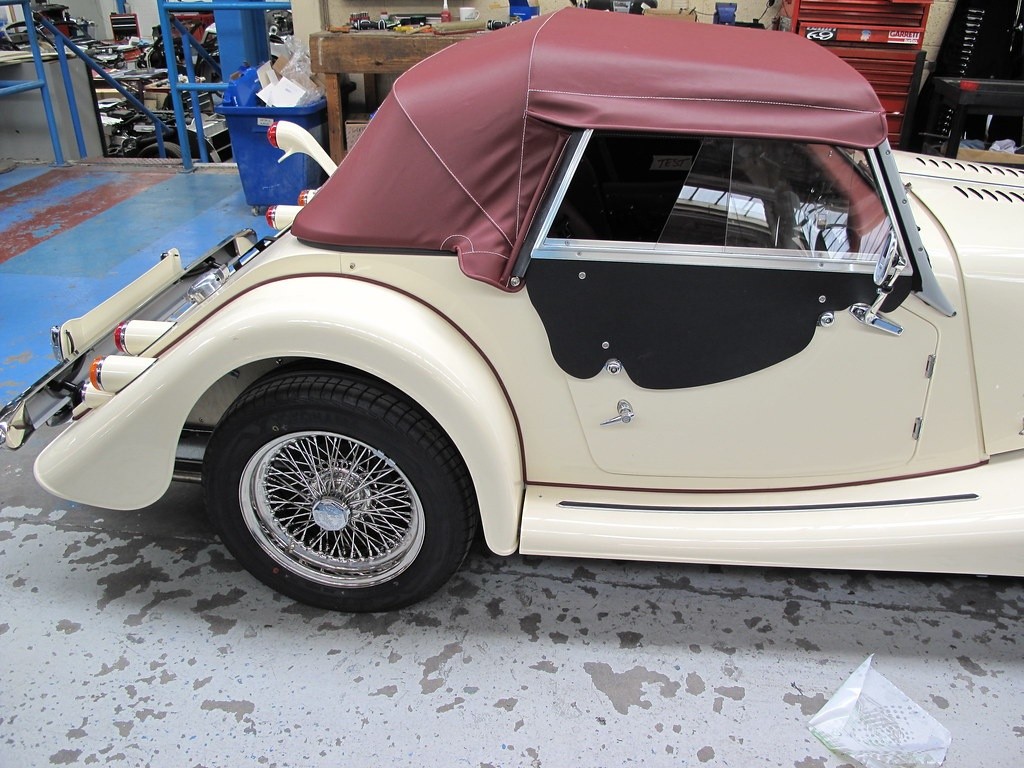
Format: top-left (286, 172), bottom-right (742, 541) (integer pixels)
top-left (213, 54), bottom-right (357, 207)
top-left (93, 88), bottom-right (122, 100)
top-left (345, 114), bottom-right (373, 152)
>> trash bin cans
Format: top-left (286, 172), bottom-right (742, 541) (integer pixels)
top-left (213, 80), bottom-right (356, 216)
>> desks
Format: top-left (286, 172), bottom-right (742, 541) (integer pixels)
top-left (309, 29), bottom-right (492, 167)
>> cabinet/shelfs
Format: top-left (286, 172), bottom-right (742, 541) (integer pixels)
top-left (0, 57), bottom-right (103, 161)
top-left (777, 0), bottom-right (933, 152)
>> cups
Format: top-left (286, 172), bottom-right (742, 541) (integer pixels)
top-left (460, 7), bottom-right (480, 21)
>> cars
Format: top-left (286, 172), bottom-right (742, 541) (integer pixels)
top-left (1, 6), bottom-right (1023, 613)
